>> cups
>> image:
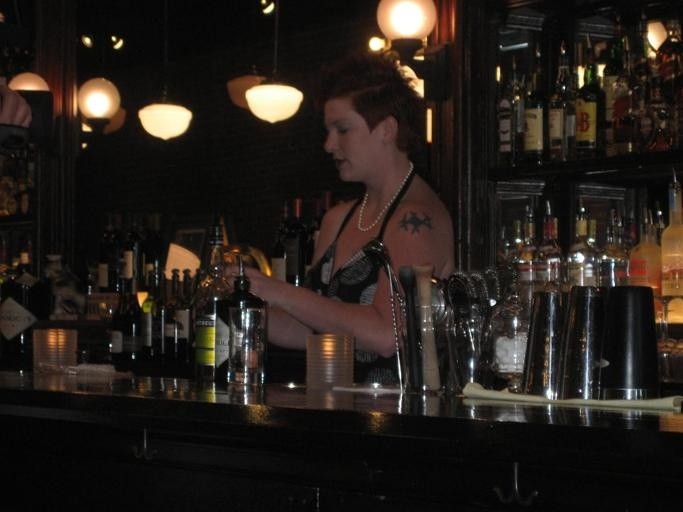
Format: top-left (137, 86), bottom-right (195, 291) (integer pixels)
top-left (307, 333), bottom-right (354, 394)
top-left (402, 295), bottom-right (525, 397)
top-left (599, 287), bottom-right (661, 402)
top-left (402, 394), bottom-right (520, 422)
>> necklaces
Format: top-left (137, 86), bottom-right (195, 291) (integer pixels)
top-left (356, 159), bottom-right (414, 232)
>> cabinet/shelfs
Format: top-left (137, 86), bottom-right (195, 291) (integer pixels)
top-left (455, 0), bottom-right (683, 339)
top-left (0, 1), bottom-right (78, 319)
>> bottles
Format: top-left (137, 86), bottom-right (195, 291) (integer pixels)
top-left (522, 402), bottom-right (663, 430)
top-left (0, 148), bottom-right (345, 382)
top-left (524, 290), bottom-right (562, 402)
top-left (559, 286), bottom-right (604, 399)
top-left (491, 16), bottom-right (683, 333)
top-left (3, 370), bottom-right (268, 409)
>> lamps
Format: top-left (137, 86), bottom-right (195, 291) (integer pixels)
top-left (7, 72), bottom-right (50, 92)
top-left (138, 0), bottom-right (194, 141)
top-left (77, 75), bottom-right (121, 144)
top-left (226, 50), bottom-right (272, 110)
top-left (375, 0), bottom-right (440, 62)
top-left (245, 1), bottom-right (304, 125)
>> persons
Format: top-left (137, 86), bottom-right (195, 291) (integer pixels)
top-left (223, 65), bottom-right (456, 385)
